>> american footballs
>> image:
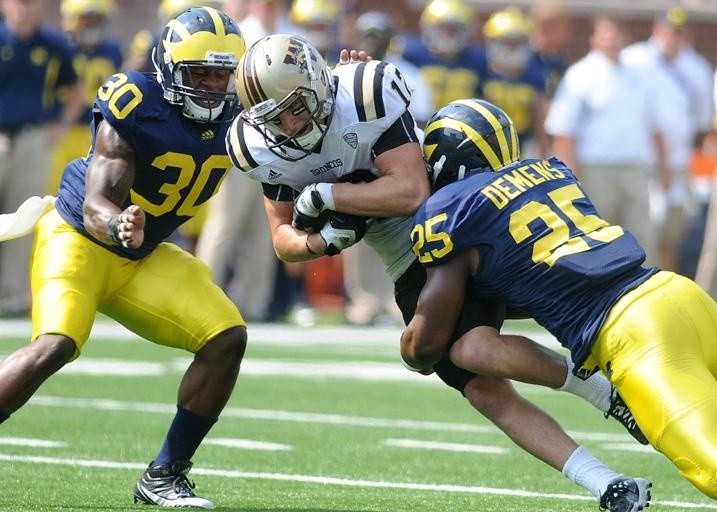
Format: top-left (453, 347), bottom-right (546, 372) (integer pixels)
top-left (327, 170), bottom-right (379, 230)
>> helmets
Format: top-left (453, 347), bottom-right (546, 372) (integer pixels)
top-left (61, 1), bottom-right (118, 46)
top-left (421, 97), bottom-right (523, 193)
top-left (161, 0), bottom-right (212, 25)
top-left (481, 11), bottom-right (533, 69)
top-left (419, 2), bottom-right (473, 60)
top-left (151, 5), bottom-right (248, 126)
top-left (290, 1), bottom-right (344, 49)
top-left (234, 32), bottom-right (335, 162)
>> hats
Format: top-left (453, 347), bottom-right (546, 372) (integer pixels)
top-left (654, 6), bottom-right (693, 30)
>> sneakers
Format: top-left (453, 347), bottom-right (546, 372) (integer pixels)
top-left (599, 477), bottom-right (653, 512)
top-left (134, 461), bottom-right (215, 510)
top-left (608, 382), bottom-right (650, 446)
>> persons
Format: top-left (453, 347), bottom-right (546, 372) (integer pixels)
top-left (399, 99), bottom-right (717, 512)
top-left (222, 35), bottom-right (653, 512)
top-left (0, 0), bottom-right (716, 321)
top-left (0, 8), bottom-right (374, 511)
